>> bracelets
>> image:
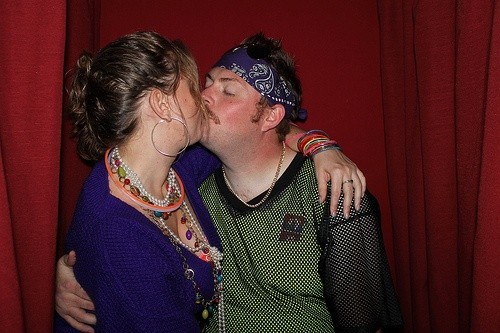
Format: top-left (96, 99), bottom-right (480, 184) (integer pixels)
top-left (296, 129), bottom-right (343, 161)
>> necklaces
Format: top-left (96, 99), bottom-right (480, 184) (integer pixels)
top-left (221, 141), bottom-right (287, 208)
top-left (104, 144), bottom-right (226, 333)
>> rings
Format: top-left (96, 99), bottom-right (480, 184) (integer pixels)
top-left (342, 180), bottom-right (354, 184)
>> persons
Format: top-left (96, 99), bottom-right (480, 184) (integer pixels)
top-left (54, 31), bottom-right (407, 333)
top-left (53, 30), bottom-right (368, 333)
